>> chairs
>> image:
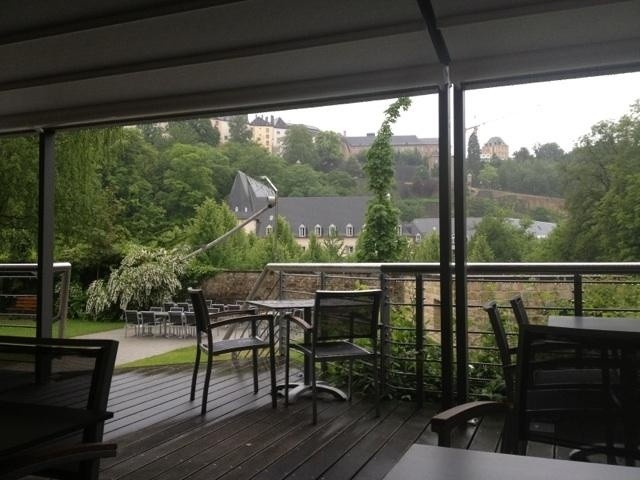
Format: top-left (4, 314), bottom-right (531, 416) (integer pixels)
top-left (283, 287), bottom-right (384, 426)
top-left (429, 293), bottom-right (640, 465)
top-left (1, 335), bottom-right (122, 479)
top-left (0, 260), bottom-right (72, 339)
top-left (185, 285), bottom-right (280, 415)
top-left (124, 300), bottom-right (242, 342)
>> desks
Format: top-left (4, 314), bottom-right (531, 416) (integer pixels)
top-left (0, 401), bottom-right (114, 460)
top-left (243, 297), bottom-right (375, 407)
top-left (380, 442), bottom-right (640, 479)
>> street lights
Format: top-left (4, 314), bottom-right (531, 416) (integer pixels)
top-left (189, 195), bottom-right (276, 257)
top-left (260, 175), bottom-right (278, 253)
